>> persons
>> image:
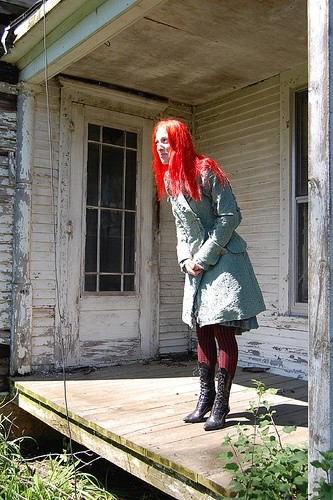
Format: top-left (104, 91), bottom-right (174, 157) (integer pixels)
top-left (151, 116), bottom-right (267, 430)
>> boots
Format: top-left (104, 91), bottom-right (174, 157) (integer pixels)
top-left (202, 367), bottom-right (235, 431)
top-left (183, 361), bottom-right (218, 424)
